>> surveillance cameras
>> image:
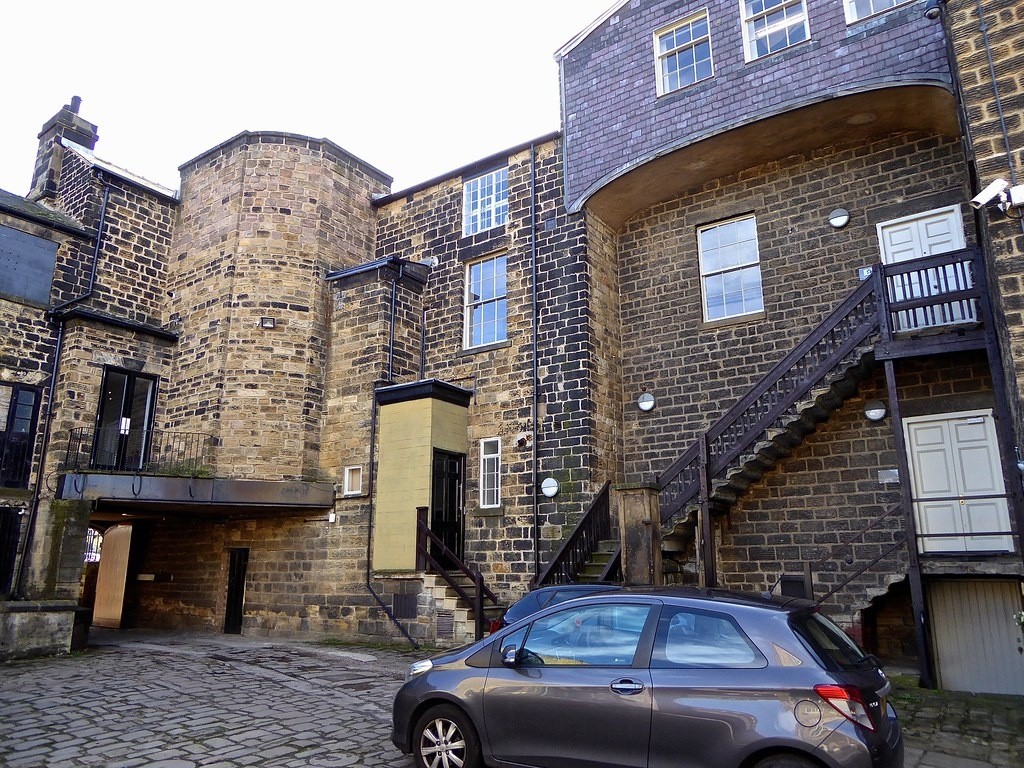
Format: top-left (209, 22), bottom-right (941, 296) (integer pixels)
top-left (968, 179), bottom-right (1008, 210)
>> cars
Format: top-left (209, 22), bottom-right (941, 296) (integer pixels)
top-left (390, 586), bottom-right (905, 768)
